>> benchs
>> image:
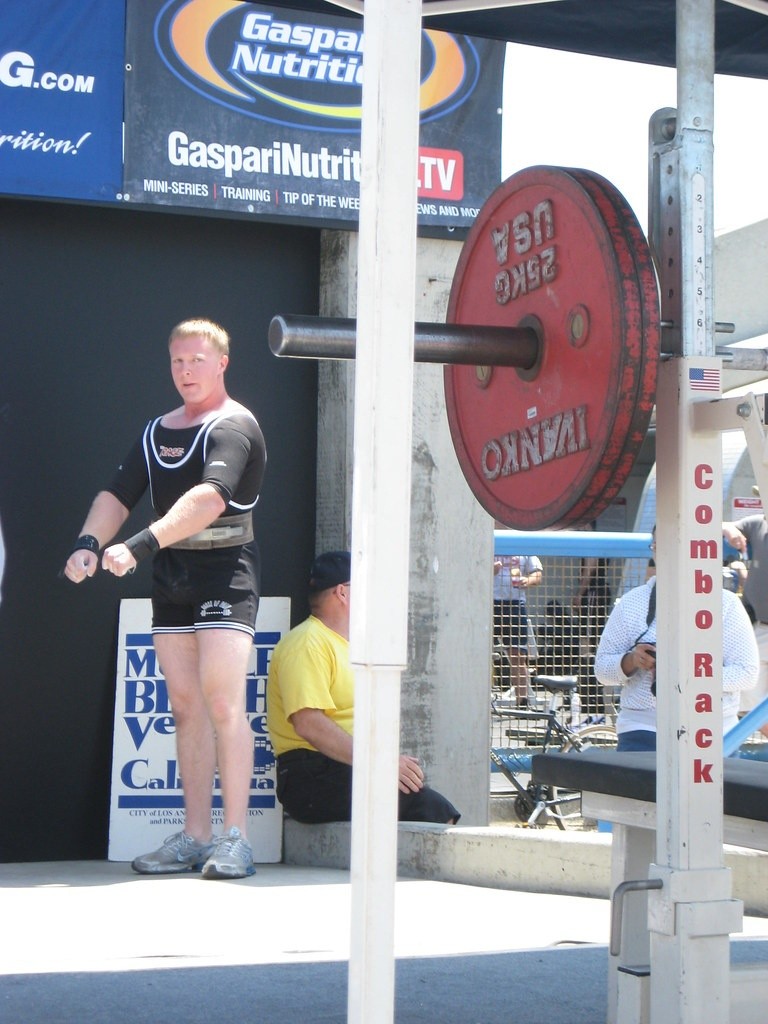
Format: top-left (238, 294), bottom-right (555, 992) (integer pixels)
top-left (530, 750), bottom-right (767, 1024)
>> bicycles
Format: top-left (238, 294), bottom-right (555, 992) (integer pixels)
top-left (488, 651), bottom-right (618, 831)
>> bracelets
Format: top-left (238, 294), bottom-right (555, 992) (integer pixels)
top-left (124, 527), bottom-right (161, 568)
top-left (65, 535), bottom-right (99, 566)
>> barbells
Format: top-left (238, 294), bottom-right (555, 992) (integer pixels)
top-left (268, 166), bottom-right (768, 531)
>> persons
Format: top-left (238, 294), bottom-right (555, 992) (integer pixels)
top-left (491, 512), bottom-right (768, 752)
top-left (64, 318), bottom-right (268, 881)
top-left (267, 551), bottom-right (462, 826)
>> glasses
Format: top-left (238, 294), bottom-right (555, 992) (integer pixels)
top-left (649, 544), bottom-right (656, 553)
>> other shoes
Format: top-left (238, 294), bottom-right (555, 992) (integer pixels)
top-left (515, 700), bottom-right (543, 720)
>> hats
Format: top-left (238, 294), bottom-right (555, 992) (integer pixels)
top-left (307, 552), bottom-right (351, 593)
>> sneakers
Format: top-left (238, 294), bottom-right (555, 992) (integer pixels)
top-left (131, 831), bottom-right (220, 874)
top-left (202, 826), bottom-right (256, 879)
